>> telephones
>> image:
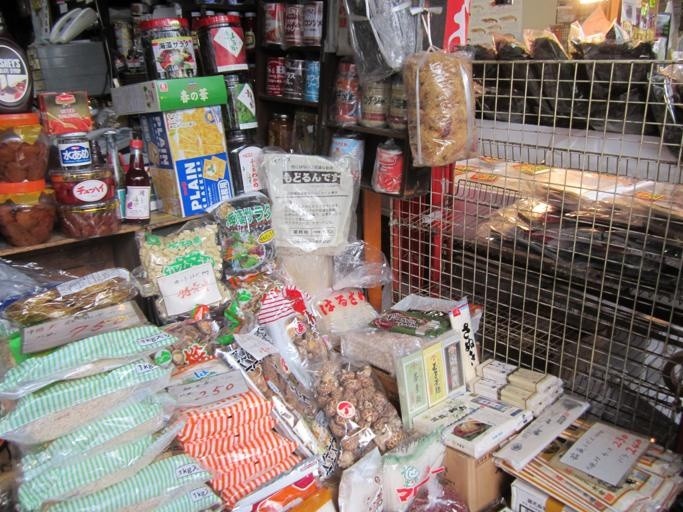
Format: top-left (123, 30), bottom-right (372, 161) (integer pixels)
top-left (49, 8), bottom-right (97, 44)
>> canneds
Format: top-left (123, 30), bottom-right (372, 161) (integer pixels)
top-left (139, 1), bottom-right (324, 198)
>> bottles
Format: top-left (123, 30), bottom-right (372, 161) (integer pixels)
top-left (0, 114), bottom-right (54, 246)
top-left (190, 11), bottom-right (257, 49)
top-left (87, 130), bottom-right (152, 224)
